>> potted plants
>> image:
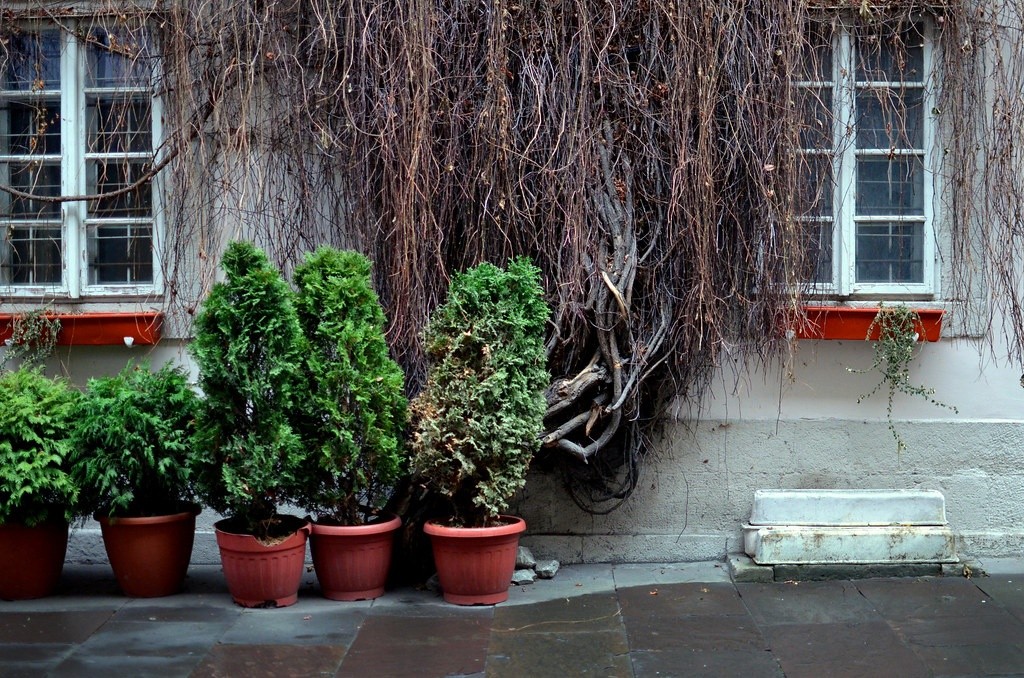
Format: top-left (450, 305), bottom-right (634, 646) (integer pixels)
top-left (0, 361), bottom-right (80, 599)
top-left (294, 248), bottom-right (407, 603)
top-left (81, 361), bottom-right (202, 599)
top-left (188, 244), bottom-right (306, 607)
top-left (412, 258), bottom-right (554, 606)
